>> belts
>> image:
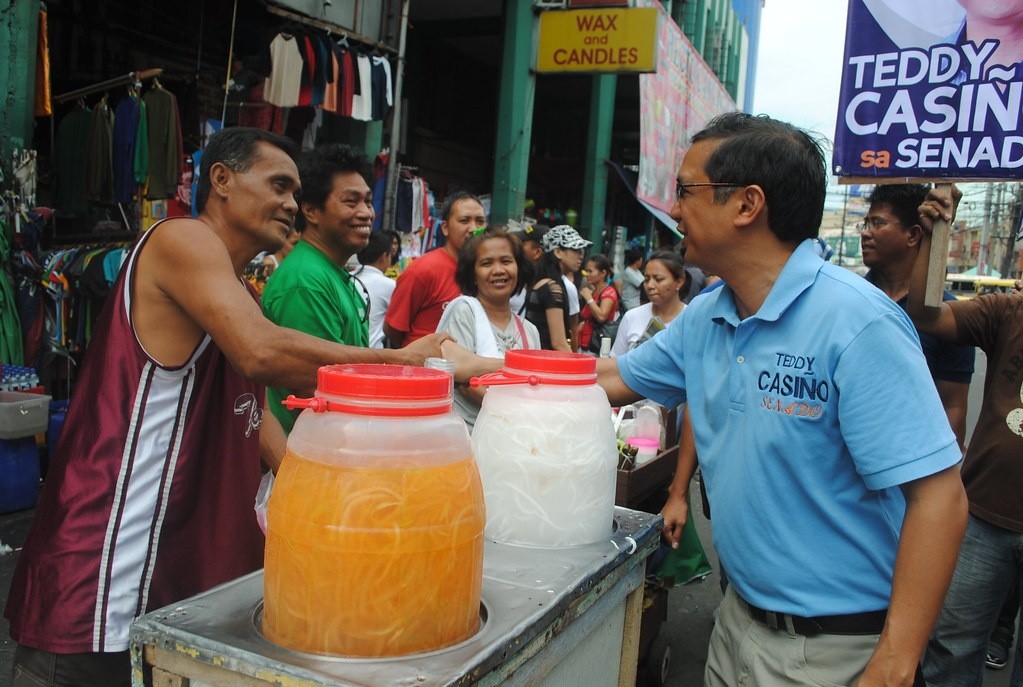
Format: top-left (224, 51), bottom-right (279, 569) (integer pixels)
top-left (747, 600), bottom-right (887, 635)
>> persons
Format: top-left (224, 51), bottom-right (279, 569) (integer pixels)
top-left (0, 127), bottom-right (457, 687)
top-left (261, 145), bottom-right (375, 435)
top-left (942, 0), bottom-right (1023, 84)
top-left (380, 230), bottom-right (400, 280)
top-left (861, 185), bottom-right (1023, 687)
top-left (260, 228), bottom-right (300, 281)
top-left (347, 236), bottom-right (397, 348)
top-left (382, 193), bottom-right (724, 591)
top-left (441, 114), bottom-right (970, 686)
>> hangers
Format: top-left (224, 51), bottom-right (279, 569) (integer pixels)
top-left (270, 16), bottom-right (393, 67)
top-left (401, 164), bottom-right (427, 185)
top-left (74, 72), bottom-right (173, 113)
top-left (35, 230), bottom-right (134, 263)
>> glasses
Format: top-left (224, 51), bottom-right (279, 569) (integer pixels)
top-left (855, 220), bottom-right (911, 232)
top-left (348, 274), bottom-right (371, 324)
top-left (676, 180), bottom-right (751, 202)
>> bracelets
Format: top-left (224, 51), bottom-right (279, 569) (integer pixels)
top-left (587, 299), bottom-right (593, 304)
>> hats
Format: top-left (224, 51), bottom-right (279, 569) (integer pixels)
top-left (516, 224), bottom-right (550, 246)
top-left (543, 225), bottom-right (594, 253)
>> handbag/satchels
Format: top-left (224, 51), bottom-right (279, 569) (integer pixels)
top-left (590, 285), bottom-right (624, 354)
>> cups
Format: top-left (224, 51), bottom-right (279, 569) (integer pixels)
top-left (423, 356), bottom-right (455, 410)
top-left (627, 436), bottom-right (660, 467)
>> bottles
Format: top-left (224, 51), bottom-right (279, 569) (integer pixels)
top-left (0, 363), bottom-right (40, 391)
top-left (468, 348), bottom-right (618, 550)
top-left (578, 270), bottom-right (587, 297)
top-left (263, 365), bottom-right (485, 659)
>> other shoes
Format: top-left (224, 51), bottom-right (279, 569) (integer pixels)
top-left (985, 653), bottom-right (1008, 668)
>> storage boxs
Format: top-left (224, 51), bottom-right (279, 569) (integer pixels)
top-left (0, 390), bottom-right (52, 438)
top-left (617, 441), bottom-right (681, 509)
